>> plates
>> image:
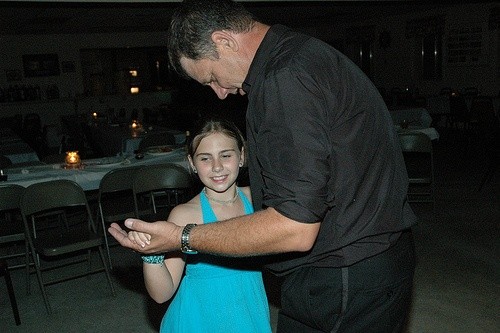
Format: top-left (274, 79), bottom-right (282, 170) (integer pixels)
top-left (95, 160), bottom-right (124, 167)
top-left (145, 148), bottom-right (176, 155)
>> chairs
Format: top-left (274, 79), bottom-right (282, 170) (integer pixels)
top-left (132, 162), bottom-right (196, 221)
top-left (376, 20), bottom-right (500, 222)
top-left (19, 179), bottom-right (118, 315)
top-left (89, 166), bottom-right (141, 276)
top-left (0, 183), bottom-right (41, 329)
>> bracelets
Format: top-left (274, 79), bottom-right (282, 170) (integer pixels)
top-left (141, 252), bottom-right (165, 263)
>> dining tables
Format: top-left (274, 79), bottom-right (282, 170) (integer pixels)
top-left (2, 44), bottom-right (192, 145)
top-left (0, 147), bottom-right (192, 206)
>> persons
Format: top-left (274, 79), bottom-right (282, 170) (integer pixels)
top-left (107, 3), bottom-right (418, 333)
top-left (132, 114), bottom-right (273, 333)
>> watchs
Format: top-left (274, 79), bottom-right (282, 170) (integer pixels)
top-left (181, 222), bottom-right (199, 254)
